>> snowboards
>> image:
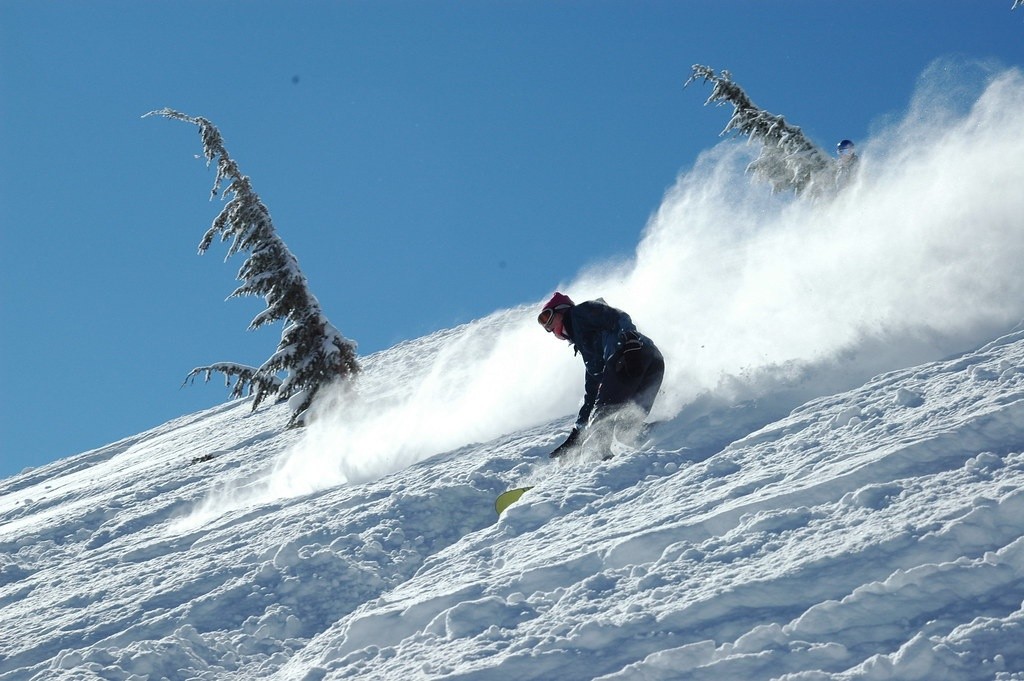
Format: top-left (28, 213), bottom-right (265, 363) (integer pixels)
top-left (494, 456), bottom-right (616, 517)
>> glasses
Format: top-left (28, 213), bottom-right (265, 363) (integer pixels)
top-left (538, 309), bottom-right (555, 332)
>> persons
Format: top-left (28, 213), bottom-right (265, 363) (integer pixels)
top-left (835, 140), bottom-right (861, 192)
top-left (537, 292), bottom-right (665, 460)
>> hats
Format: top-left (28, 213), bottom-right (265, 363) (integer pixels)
top-left (542, 292), bottom-right (575, 309)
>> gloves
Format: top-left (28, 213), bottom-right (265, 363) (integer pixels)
top-left (549, 428), bottom-right (579, 459)
top-left (606, 330), bottom-right (643, 371)
